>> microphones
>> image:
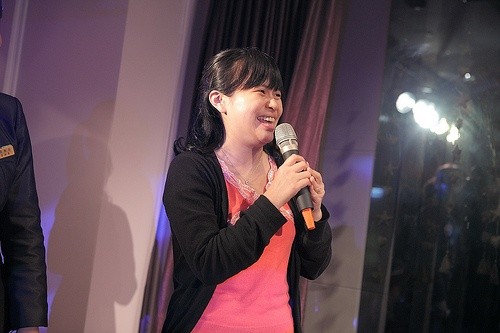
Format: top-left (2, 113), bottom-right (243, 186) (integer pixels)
top-left (273, 122), bottom-right (316, 230)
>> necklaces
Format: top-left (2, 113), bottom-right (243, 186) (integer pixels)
top-left (219, 144), bottom-right (263, 185)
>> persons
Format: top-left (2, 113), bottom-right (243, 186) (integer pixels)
top-left (1, 91), bottom-right (48, 333)
top-left (158, 46), bottom-right (334, 333)
